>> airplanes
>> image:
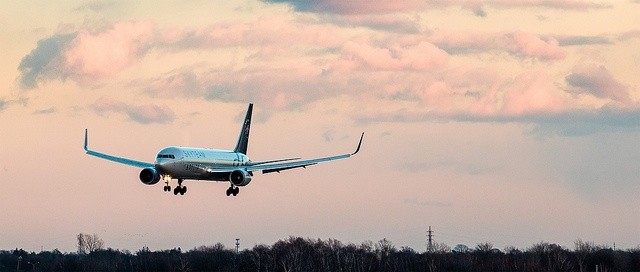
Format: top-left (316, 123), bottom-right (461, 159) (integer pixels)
top-left (81, 103), bottom-right (367, 196)
